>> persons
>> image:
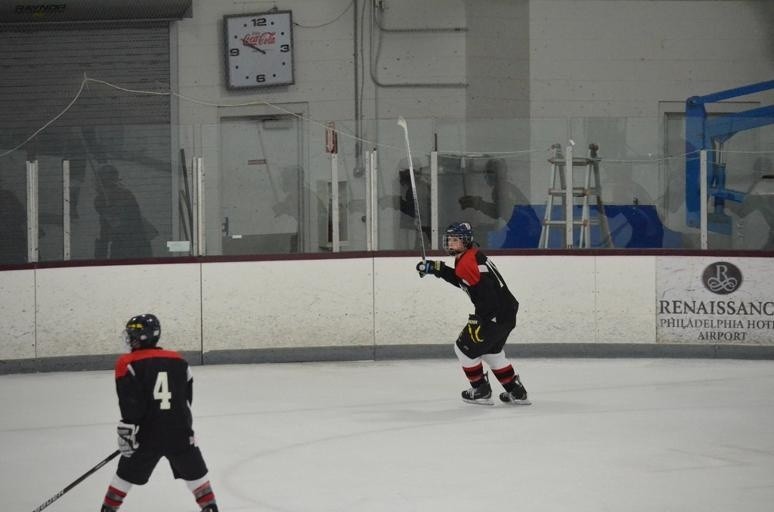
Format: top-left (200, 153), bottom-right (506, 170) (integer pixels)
top-left (0, 153), bottom-right (774, 262)
top-left (96, 312), bottom-right (218, 511)
top-left (417, 218), bottom-right (529, 403)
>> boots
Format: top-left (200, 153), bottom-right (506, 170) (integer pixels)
top-left (100, 484), bottom-right (128, 512)
top-left (461, 366), bottom-right (527, 404)
top-left (188, 480), bottom-right (219, 511)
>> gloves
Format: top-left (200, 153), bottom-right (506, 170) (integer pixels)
top-left (415, 259), bottom-right (441, 278)
top-left (116, 420), bottom-right (140, 459)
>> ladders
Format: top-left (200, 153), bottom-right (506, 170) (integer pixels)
top-left (538, 143), bottom-right (613, 249)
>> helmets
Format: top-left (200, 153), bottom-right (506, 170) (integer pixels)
top-left (126, 314), bottom-right (161, 350)
top-left (442, 222), bottom-right (474, 256)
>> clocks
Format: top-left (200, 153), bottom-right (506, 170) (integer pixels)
top-left (223, 11), bottom-right (295, 91)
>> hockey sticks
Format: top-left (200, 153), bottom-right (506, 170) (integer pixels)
top-left (397, 117), bottom-right (427, 263)
